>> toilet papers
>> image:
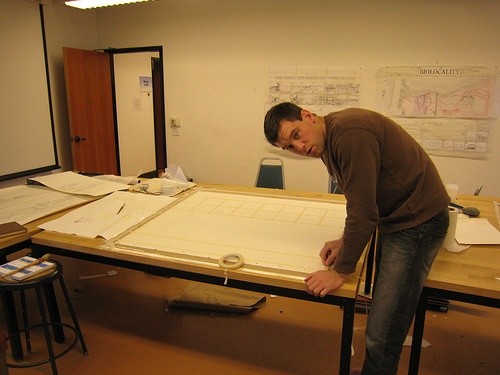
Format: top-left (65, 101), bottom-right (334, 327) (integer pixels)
top-left (442, 205), bottom-right (458, 249)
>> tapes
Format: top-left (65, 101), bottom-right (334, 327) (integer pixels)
top-left (218, 252), bottom-right (244, 270)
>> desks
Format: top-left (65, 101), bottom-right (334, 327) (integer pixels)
top-left (408, 194), bottom-right (500, 375)
top-left (0, 171), bottom-right (377, 375)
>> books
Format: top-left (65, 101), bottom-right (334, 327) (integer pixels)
top-left (0, 221), bottom-right (28, 239)
top-left (0, 256), bottom-right (57, 283)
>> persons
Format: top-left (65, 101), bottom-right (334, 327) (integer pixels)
top-left (263, 102), bottom-right (451, 375)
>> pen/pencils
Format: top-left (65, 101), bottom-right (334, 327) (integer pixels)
top-left (117, 202), bottom-right (126, 214)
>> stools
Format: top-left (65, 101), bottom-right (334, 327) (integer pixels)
top-left (0, 259), bottom-right (89, 375)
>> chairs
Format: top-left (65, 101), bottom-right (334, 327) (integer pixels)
top-left (255, 157), bottom-right (286, 190)
